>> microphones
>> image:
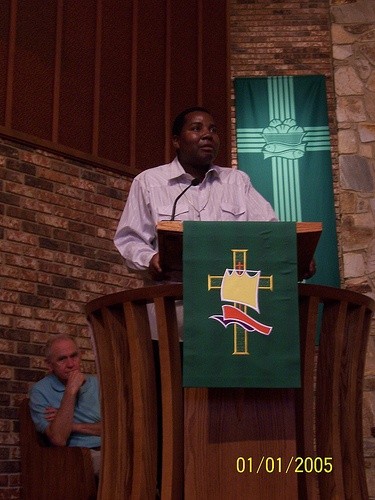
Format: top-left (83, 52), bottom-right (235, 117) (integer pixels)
top-left (171, 178), bottom-right (200, 221)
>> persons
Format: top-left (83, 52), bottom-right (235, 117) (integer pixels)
top-left (114, 107), bottom-right (317, 500)
top-left (29, 335), bottom-right (104, 487)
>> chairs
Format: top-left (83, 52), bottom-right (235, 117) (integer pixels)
top-left (21, 397), bottom-right (97, 500)
